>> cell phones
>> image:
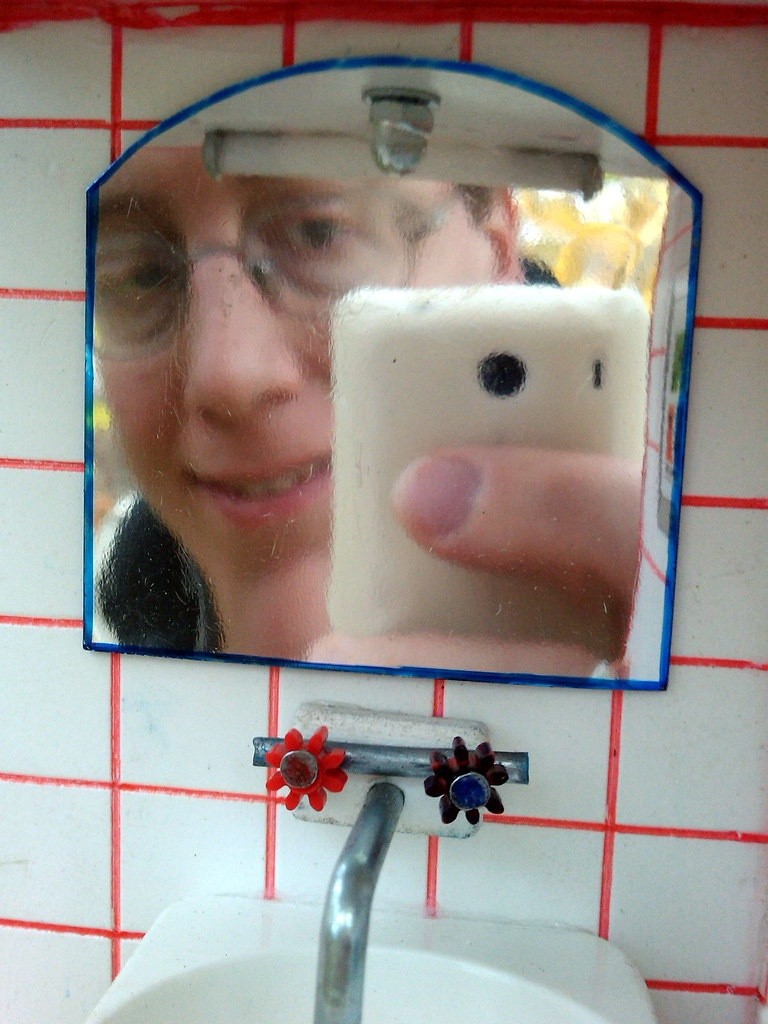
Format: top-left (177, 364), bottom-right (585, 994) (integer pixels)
top-left (330, 285), bottom-right (649, 662)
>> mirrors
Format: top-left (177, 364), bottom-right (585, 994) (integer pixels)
top-left (84, 52), bottom-right (702, 691)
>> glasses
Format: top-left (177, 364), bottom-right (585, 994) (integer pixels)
top-left (88, 185), bottom-right (471, 360)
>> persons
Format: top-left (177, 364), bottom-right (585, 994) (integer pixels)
top-left (94, 145), bottom-right (638, 677)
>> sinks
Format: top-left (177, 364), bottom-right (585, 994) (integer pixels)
top-left (84, 895), bottom-right (660, 1023)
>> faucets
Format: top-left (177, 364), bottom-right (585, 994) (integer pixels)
top-left (312, 782), bottom-right (408, 1024)
